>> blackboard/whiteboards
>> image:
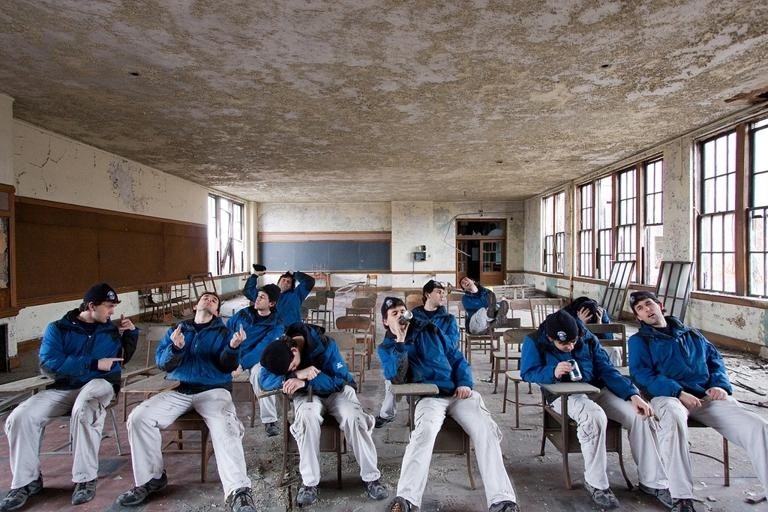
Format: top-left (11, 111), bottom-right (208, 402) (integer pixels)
top-left (258, 239), bottom-right (392, 273)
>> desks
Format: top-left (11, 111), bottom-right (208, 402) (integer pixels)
top-left (312, 273), bottom-right (331, 287)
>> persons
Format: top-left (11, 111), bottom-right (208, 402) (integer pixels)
top-left (116, 291), bottom-right (258, 512)
top-left (374, 279), bottom-right (461, 428)
top-left (560, 296), bottom-right (622, 367)
top-left (0, 282), bottom-right (139, 511)
top-left (377, 297), bottom-right (520, 512)
top-left (225, 283), bottom-right (285, 437)
top-left (256, 322), bottom-right (388, 506)
top-left (628, 290), bottom-right (768, 511)
top-left (520, 308), bottom-right (674, 510)
top-left (242, 270), bottom-right (314, 333)
top-left (458, 275), bottom-right (509, 336)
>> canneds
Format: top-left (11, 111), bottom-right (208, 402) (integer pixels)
top-left (568, 359), bottom-right (583, 381)
top-left (399, 309), bottom-right (413, 325)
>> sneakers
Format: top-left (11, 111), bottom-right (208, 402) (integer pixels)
top-left (118, 471), bottom-right (168, 507)
top-left (71, 478), bottom-right (98, 503)
top-left (365, 479), bottom-right (387, 500)
top-left (486, 291), bottom-right (509, 327)
top-left (229, 486), bottom-right (257, 511)
top-left (386, 496), bottom-right (419, 512)
top-left (295, 485), bottom-right (321, 508)
top-left (639, 482), bottom-right (694, 509)
top-left (1, 473), bottom-right (43, 511)
top-left (374, 416), bottom-right (398, 429)
top-left (583, 479), bottom-right (620, 508)
top-left (264, 421), bottom-right (281, 437)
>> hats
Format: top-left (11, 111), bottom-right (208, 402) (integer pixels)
top-left (545, 310), bottom-right (578, 341)
top-left (630, 291), bottom-right (655, 306)
top-left (84, 282), bottom-right (119, 301)
top-left (382, 297), bottom-right (403, 314)
top-left (423, 281), bottom-right (446, 290)
top-left (263, 340), bottom-right (289, 374)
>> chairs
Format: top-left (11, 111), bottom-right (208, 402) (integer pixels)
top-left (366, 274), bottom-right (378, 296)
top-left (138, 280), bottom-right (193, 323)
